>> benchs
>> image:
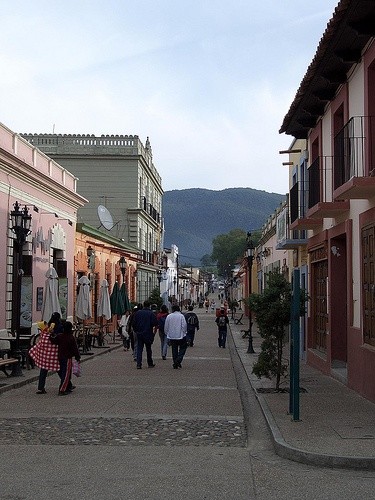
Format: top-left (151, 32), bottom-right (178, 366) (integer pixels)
top-left (0, 358), bottom-right (25, 388)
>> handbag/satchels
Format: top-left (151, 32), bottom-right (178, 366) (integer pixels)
top-left (159, 317), bottom-right (166, 331)
top-left (72, 359), bottom-right (81, 377)
top-left (118, 326), bottom-right (124, 335)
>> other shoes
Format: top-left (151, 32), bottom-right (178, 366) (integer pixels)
top-left (132, 352), bottom-right (134, 355)
top-left (137, 365), bottom-right (141, 369)
top-left (134, 357), bottom-right (137, 361)
top-left (162, 354), bottom-right (166, 360)
top-left (36, 387), bottom-right (47, 394)
top-left (223, 346), bottom-right (225, 348)
top-left (58, 390), bottom-right (68, 395)
top-left (71, 386), bottom-right (76, 389)
top-left (148, 364), bottom-right (155, 368)
top-left (219, 344), bottom-right (221, 347)
top-left (173, 364), bottom-right (178, 369)
top-left (187, 342), bottom-right (193, 347)
top-left (124, 349), bottom-right (127, 352)
top-left (177, 362), bottom-right (182, 368)
top-left (66, 389), bottom-right (73, 392)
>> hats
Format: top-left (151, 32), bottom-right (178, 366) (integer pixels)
top-left (38, 320), bottom-right (47, 330)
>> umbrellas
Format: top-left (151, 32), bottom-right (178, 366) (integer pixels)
top-left (75, 276), bottom-right (91, 352)
top-left (42, 267), bottom-right (63, 324)
top-left (110, 281), bottom-right (130, 343)
top-left (96, 280), bottom-right (112, 347)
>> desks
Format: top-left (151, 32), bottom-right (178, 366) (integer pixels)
top-left (0, 323), bottom-right (120, 387)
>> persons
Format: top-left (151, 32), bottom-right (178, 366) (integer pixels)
top-left (119, 292), bottom-right (229, 369)
top-left (28, 312), bottom-right (80, 396)
